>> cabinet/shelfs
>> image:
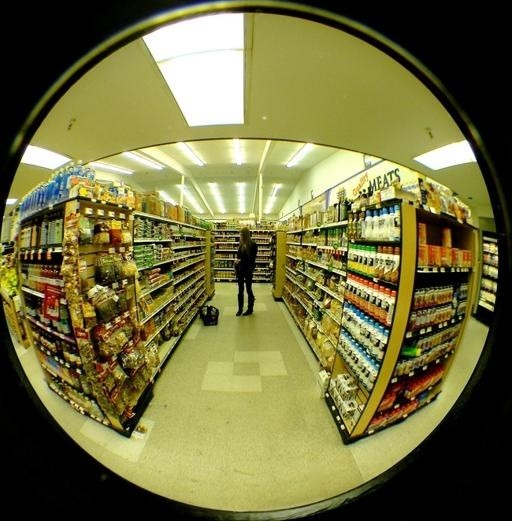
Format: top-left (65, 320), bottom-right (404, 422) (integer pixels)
top-left (15, 198), bottom-right (154, 438)
top-left (210, 227), bottom-right (274, 282)
top-left (278, 221), bottom-right (349, 367)
top-left (478, 231), bottom-right (500, 316)
top-left (131, 211), bottom-right (210, 378)
top-left (325, 189), bottom-right (478, 446)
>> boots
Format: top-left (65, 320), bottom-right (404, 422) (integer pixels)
top-left (242, 296), bottom-right (256, 315)
top-left (236, 295), bottom-right (243, 316)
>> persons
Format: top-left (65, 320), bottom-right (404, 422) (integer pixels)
top-left (233, 229), bottom-right (258, 315)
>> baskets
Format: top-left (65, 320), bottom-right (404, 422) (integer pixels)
top-left (199, 305), bottom-right (219, 326)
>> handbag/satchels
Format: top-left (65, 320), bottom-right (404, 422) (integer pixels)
top-left (234, 262), bottom-right (244, 278)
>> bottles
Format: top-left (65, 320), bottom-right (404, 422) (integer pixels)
top-left (337, 329), bottom-right (380, 392)
top-left (23, 296), bottom-right (72, 336)
top-left (344, 274), bottom-right (397, 327)
top-left (408, 303), bottom-right (452, 330)
top-left (327, 228), bottom-right (346, 247)
top-left (414, 284), bottom-right (454, 310)
top-left (345, 244), bottom-right (401, 283)
top-left (21, 263), bottom-right (65, 295)
top-left (335, 195), bottom-right (354, 222)
top-left (341, 302), bottom-right (389, 362)
top-left (347, 205), bottom-right (401, 241)
top-left (19, 159), bottom-right (95, 220)
top-left (459, 283), bottom-right (469, 299)
top-left (30, 325), bottom-right (83, 372)
